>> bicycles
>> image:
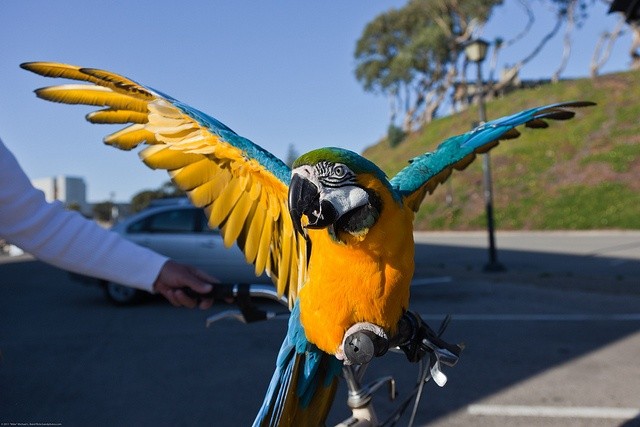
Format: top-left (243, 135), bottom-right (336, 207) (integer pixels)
top-left (185, 281), bottom-right (463, 423)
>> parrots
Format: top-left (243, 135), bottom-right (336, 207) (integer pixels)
top-left (18, 61), bottom-right (598, 427)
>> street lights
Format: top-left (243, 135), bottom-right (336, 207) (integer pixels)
top-left (462, 36), bottom-right (506, 270)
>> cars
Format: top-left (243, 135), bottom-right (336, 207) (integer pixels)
top-left (104, 196), bottom-right (276, 302)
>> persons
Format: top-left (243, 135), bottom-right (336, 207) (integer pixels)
top-left (1, 135), bottom-right (224, 310)
